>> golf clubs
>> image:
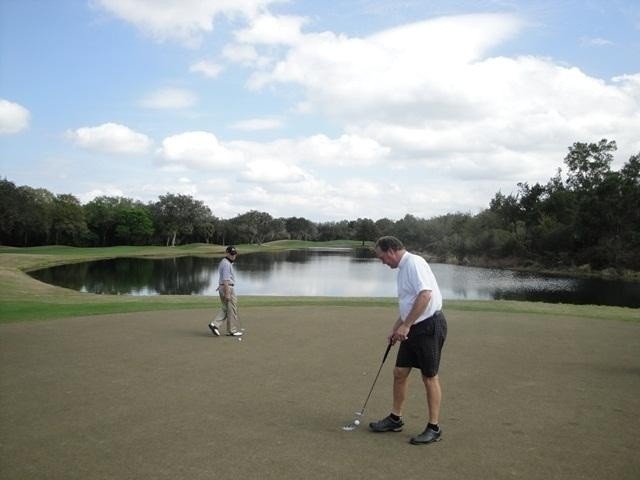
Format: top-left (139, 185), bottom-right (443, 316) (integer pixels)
top-left (341, 334), bottom-right (395, 432)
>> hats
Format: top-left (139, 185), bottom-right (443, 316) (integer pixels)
top-left (226, 247), bottom-right (237, 255)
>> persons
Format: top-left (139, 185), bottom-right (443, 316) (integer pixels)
top-left (368, 235), bottom-right (448, 445)
top-left (209, 246), bottom-right (243, 337)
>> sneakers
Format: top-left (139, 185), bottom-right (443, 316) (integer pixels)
top-left (209, 323), bottom-right (220, 336)
top-left (370, 416), bottom-right (404, 432)
top-left (226, 332), bottom-right (243, 336)
top-left (411, 427), bottom-right (442, 445)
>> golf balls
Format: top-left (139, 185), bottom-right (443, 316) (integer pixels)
top-left (238, 337), bottom-right (242, 341)
top-left (353, 419), bottom-right (360, 426)
